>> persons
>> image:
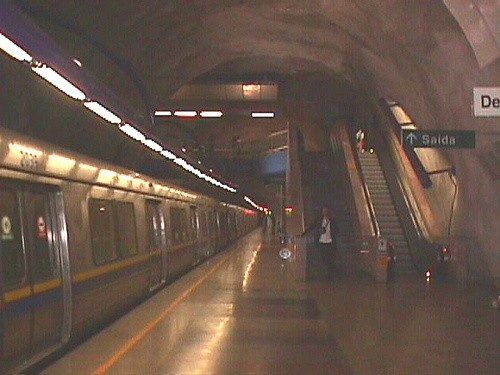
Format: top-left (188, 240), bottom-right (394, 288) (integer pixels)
top-left (262, 213), bottom-right (281, 234)
top-left (356, 129), bottom-right (365, 152)
top-left (298, 204), bottom-right (339, 280)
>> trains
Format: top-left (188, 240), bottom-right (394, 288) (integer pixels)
top-left (0, 127), bottom-right (265, 375)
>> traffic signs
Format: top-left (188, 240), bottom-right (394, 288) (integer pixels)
top-left (400, 129), bottom-right (476, 150)
